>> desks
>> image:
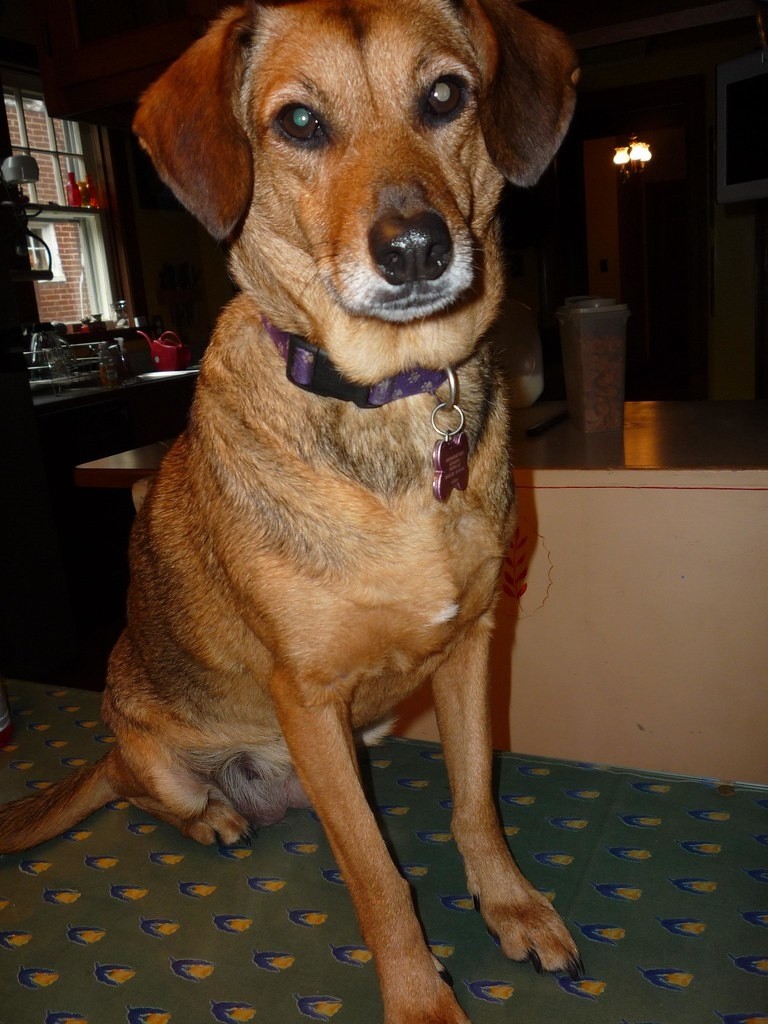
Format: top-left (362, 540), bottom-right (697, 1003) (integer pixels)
top-left (0, 674), bottom-right (768, 1024)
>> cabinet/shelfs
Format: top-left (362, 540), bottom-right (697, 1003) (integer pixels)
top-left (1, 372), bottom-right (198, 688)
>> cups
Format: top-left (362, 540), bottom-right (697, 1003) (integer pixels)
top-left (557, 297), bottom-right (630, 435)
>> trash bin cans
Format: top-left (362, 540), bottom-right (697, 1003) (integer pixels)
top-left (555, 294), bottom-right (631, 434)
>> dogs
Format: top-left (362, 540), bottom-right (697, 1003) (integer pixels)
top-left (2, 1), bottom-right (609, 1023)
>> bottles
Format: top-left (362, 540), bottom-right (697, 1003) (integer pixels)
top-left (66, 172), bottom-right (100, 208)
top-left (97, 337), bottom-right (129, 389)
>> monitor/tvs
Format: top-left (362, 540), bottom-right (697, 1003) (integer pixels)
top-left (716, 50), bottom-right (768, 203)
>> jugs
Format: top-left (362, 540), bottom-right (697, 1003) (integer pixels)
top-left (136, 330), bottom-right (191, 372)
